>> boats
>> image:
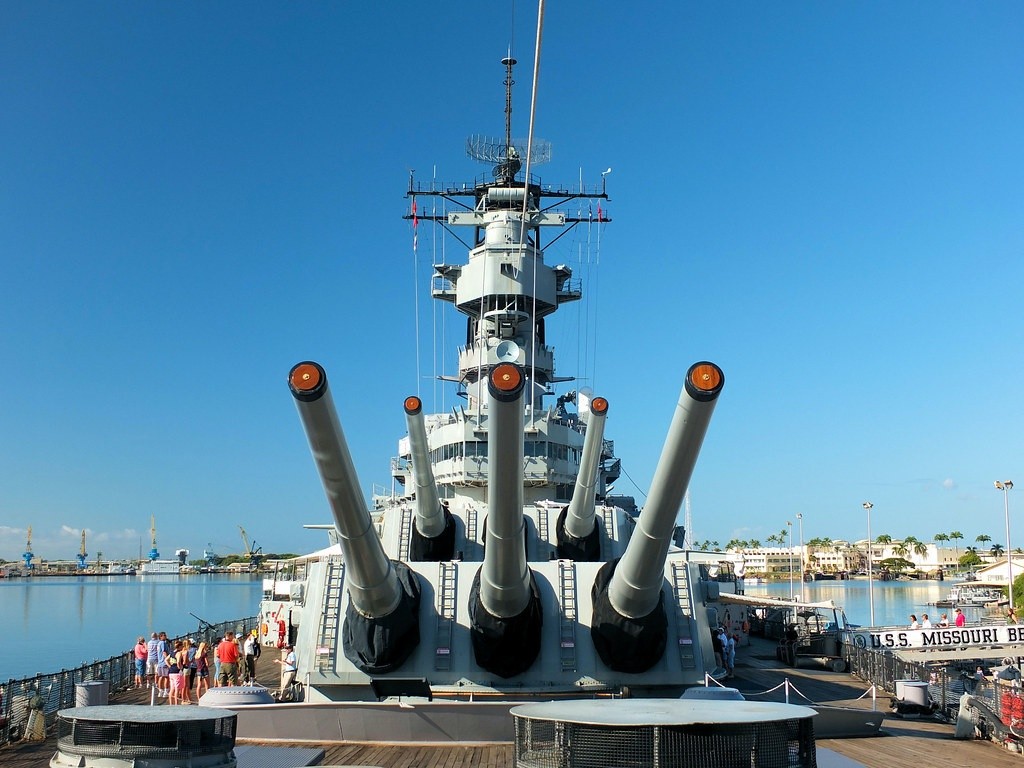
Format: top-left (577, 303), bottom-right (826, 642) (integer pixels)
top-left (936, 585), bottom-right (1009, 610)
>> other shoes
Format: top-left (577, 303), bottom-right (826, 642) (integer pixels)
top-left (275, 698), bottom-right (284, 703)
top-left (181, 700), bottom-right (191, 705)
top-left (147, 685), bottom-right (151, 689)
top-left (162, 694), bottom-right (169, 698)
top-left (158, 692), bottom-right (163, 697)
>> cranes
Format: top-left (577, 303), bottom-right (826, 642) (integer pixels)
top-left (239, 525), bottom-right (261, 561)
top-left (75, 530), bottom-right (89, 572)
top-left (22, 525), bottom-right (35, 569)
top-left (148, 514), bottom-right (160, 561)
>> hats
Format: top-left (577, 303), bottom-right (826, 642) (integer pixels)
top-left (284, 644), bottom-right (293, 650)
top-left (718, 627), bottom-right (724, 633)
top-left (189, 639), bottom-right (196, 645)
top-left (151, 633), bottom-right (160, 638)
top-left (235, 633), bottom-right (243, 641)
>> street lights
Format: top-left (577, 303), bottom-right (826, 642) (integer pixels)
top-left (862, 502), bottom-right (874, 628)
top-left (993, 480), bottom-right (1014, 612)
top-left (795, 513), bottom-right (806, 613)
top-left (786, 521), bottom-right (794, 602)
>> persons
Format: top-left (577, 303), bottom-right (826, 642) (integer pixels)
top-left (935, 613), bottom-right (949, 651)
top-left (955, 609), bottom-right (967, 651)
top-left (273, 645), bottom-right (297, 702)
top-left (919, 614), bottom-right (934, 652)
top-left (134, 632), bottom-right (261, 705)
top-left (910, 615), bottom-right (920, 630)
top-left (710, 628), bottom-right (736, 678)
top-left (1007, 608), bottom-right (1017, 648)
top-left (977, 667), bottom-right (985, 680)
